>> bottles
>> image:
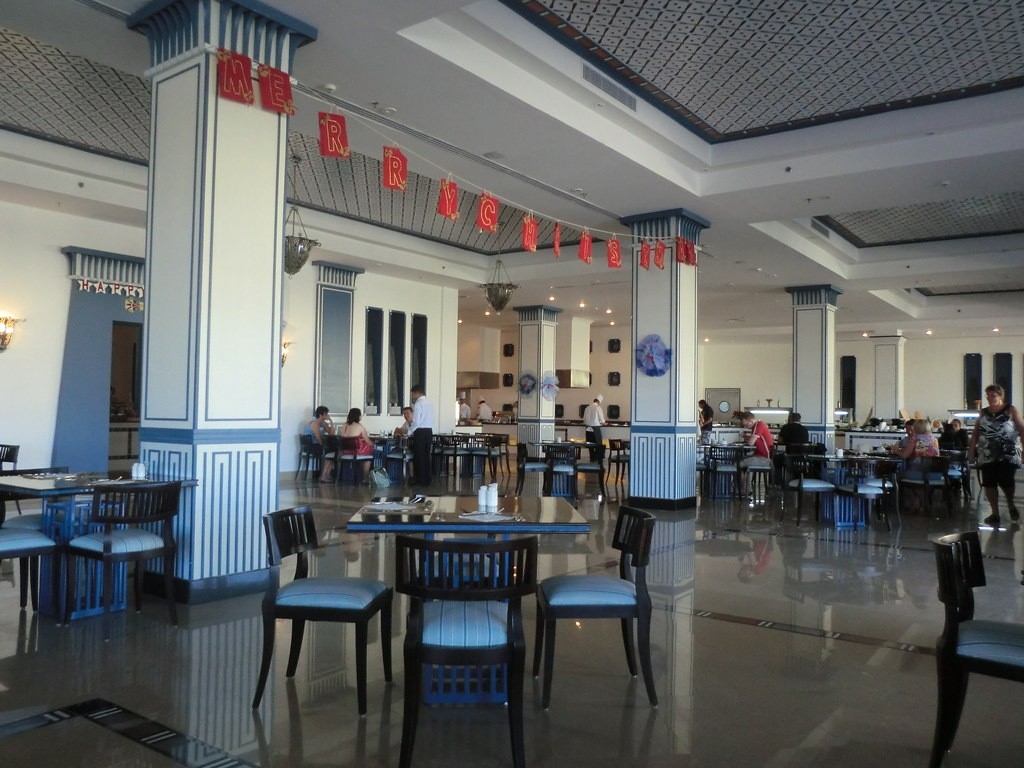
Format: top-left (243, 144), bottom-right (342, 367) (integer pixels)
top-left (557, 436), bottom-right (561, 443)
top-left (836, 449), bottom-right (844, 458)
top-left (477, 483), bottom-right (498, 513)
top-left (131, 462), bottom-right (145, 480)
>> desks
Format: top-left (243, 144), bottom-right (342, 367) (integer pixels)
top-left (697, 441), bottom-right (757, 501)
top-left (804, 452), bottom-right (891, 528)
top-left (457, 438), bottom-right (486, 478)
top-left (365, 435), bottom-right (401, 485)
top-left (530, 440), bottom-right (595, 500)
top-left (347, 493), bottom-right (592, 706)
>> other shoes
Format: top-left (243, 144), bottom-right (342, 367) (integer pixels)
top-left (984, 514), bottom-right (1000, 522)
top-left (741, 481), bottom-right (753, 496)
top-left (319, 476), bottom-right (332, 483)
top-left (1008, 506), bottom-right (1020, 521)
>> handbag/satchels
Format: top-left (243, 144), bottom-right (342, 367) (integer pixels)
top-left (368, 466), bottom-right (392, 488)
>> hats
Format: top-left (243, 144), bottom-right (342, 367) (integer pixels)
top-left (460, 392), bottom-right (465, 399)
top-left (479, 396), bottom-right (485, 403)
top-left (594, 394), bottom-right (603, 404)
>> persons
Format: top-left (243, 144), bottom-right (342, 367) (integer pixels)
top-left (303, 406), bottom-right (335, 482)
top-left (968, 385), bottom-right (1024, 523)
top-left (583, 394), bottom-right (605, 465)
top-left (698, 400), bottom-right (713, 444)
top-left (394, 385), bottom-right (433, 486)
top-left (459, 391), bottom-right (492, 420)
top-left (774, 413), bottom-right (808, 489)
top-left (735, 411), bottom-right (773, 495)
top-left (890, 419), bottom-right (968, 514)
top-left (341, 408), bottom-right (374, 485)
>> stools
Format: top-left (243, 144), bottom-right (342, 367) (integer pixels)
top-left (0, 526), bottom-right (63, 627)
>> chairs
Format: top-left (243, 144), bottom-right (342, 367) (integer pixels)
top-left (927, 531), bottom-right (1024, 767)
top-left (391, 534), bottom-right (538, 768)
top-left (0, 491), bottom-right (45, 611)
top-left (533, 505), bottom-right (658, 712)
top-left (63, 479), bottom-right (183, 641)
top-left (251, 506), bottom-right (394, 720)
top-left (295, 431), bottom-right (983, 529)
top-left (1, 446), bottom-right (23, 516)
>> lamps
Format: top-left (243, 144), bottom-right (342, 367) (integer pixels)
top-left (484, 221), bottom-right (519, 313)
top-left (0, 318), bottom-right (15, 351)
top-left (280, 155), bottom-right (320, 279)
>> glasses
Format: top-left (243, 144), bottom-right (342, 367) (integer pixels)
top-left (985, 394), bottom-right (998, 398)
